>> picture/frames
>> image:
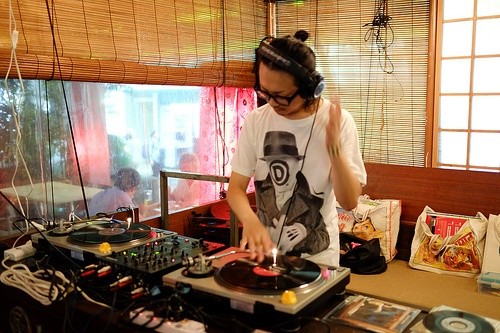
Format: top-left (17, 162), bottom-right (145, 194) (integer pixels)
top-left (331, 295), bottom-right (422, 333)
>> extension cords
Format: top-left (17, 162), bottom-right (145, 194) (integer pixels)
top-left (130, 307), bottom-right (205, 333)
top-left (4, 244), bottom-right (36, 261)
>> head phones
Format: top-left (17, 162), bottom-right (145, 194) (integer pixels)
top-left (257, 41), bottom-right (325, 100)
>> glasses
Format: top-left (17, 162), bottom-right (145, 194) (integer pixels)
top-left (253, 83), bottom-right (300, 106)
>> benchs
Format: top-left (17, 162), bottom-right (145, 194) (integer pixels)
top-left (336, 162), bottom-right (500, 320)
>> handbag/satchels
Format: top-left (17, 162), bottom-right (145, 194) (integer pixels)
top-left (339, 231), bottom-right (388, 273)
top-left (478, 213), bottom-right (500, 288)
top-left (334, 194), bottom-right (403, 263)
top-left (408, 205), bottom-right (488, 278)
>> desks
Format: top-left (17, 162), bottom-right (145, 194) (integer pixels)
top-left (0, 182), bottom-right (104, 233)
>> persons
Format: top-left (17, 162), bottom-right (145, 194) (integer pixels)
top-left (86, 168), bottom-right (140, 220)
top-left (351, 215), bottom-right (384, 241)
top-left (225, 30), bottom-right (367, 269)
top-left (167, 153), bottom-right (200, 209)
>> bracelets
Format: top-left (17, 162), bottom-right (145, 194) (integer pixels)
top-left (327, 142), bottom-right (342, 158)
